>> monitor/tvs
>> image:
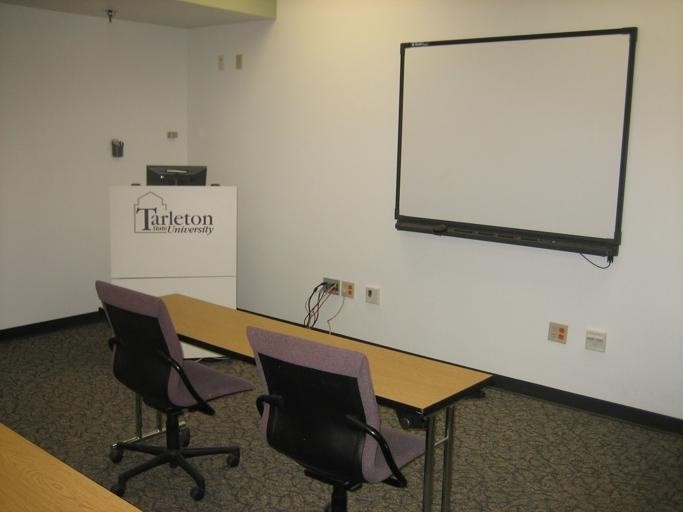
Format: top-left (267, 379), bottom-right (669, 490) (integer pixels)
top-left (147, 166), bottom-right (206, 185)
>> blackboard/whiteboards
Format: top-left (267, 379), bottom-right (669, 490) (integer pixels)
top-left (394, 27), bottom-right (636, 256)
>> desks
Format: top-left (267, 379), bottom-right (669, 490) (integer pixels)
top-left (0, 422), bottom-right (144, 512)
top-left (109, 292), bottom-right (494, 511)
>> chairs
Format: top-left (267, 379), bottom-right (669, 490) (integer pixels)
top-left (245, 325), bottom-right (426, 512)
top-left (95, 280), bottom-right (257, 501)
